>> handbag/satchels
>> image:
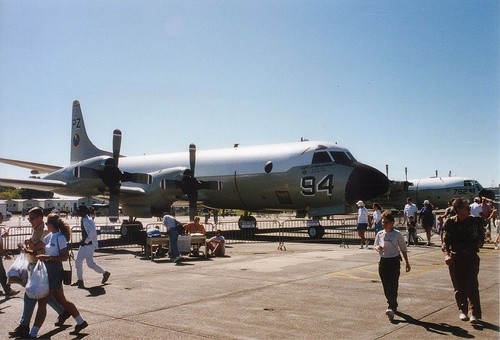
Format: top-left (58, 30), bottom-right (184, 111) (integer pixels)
top-left (63, 270), bottom-right (72, 285)
top-left (399, 253), bottom-right (403, 261)
top-left (174, 218), bottom-right (185, 236)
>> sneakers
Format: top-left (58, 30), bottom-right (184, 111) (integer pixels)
top-left (9, 324), bottom-right (29, 335)
top-left (15, 334), bottom-right (37, 340)
top-left (459, 310), bottom-right (467, 319)
top-left (365, 240), bottom-right (369, 247)
top-left (71, 280), bottom-right (84, 286)
top-left (174, 255), bottom-right (182, 263)
top-left (470, 315), bottom-right (478, 323)
top-left (69, 321), bottom-right (88, 335)
top-left (55, 311), bottom-right (71, 326)
top-left (101, 271), bottom-right (111, 284)
top-left (386, 309), bottom-right (394, 322)
top-left (359, 246), bottom-right (363, 249)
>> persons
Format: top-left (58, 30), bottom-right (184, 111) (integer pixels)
top-left (14, 212), bottom-right (88, 340)
top-left (9, 207), bottom-right (71, 336)
top-left (438, 196), bottom-right (500, 251)
top-left (211, 209), bottom-right (219, 224)
top-left (22, 208), bottom-right (25, 220)
top-left (374, 210), bottom-right (411, 318)
top-left (91, 207), bottom-right (95, 220)
top-left (70, 208), bottom-right (73, 219)
top-left (0, 211), bottom-right (21, 296)
top-left (369, 203), bottom-right (383, 237)
top-left (66, 207), bottom-right (70, 218)
top-left (159, 210), bottom-right (163, 221)
top-left (6, 208), bottom-right (12, 217)
top-left (183, 216), bottom-right (206, 256)
top-left (444, 198), bottom-right (484, 323)
top-left (402, 197), bottom-right (418, 243)
top-left (71, 206), bottom-right (111, 286)
top-left (162, 212), bottom-right (182, 265)
top-left (418, 200), bottom-right (435, 246)
top-left (205, 209), bottom-right (210, 224)
top-left (406, 216), bottom-right (418, 246)
top-left (356, 200), bottom-right (372, 249)
top-left (144, 226), bottom-right (162, 256)
top-left (117, 208), bottom-right (121, 224)
top-left (205, 229), bottom-right (226, 259)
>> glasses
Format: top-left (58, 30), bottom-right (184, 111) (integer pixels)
top-left (45, 222), bottom-right (49, 226)
top-left (27, 215), bottom-right (39, 221)
top-left (463, 206), bottom-right (471, 211)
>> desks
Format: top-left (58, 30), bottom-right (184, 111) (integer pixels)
top-left (147, 233), bottom-right (207, 261)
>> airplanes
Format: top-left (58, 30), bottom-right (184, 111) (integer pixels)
top-left (0, 99), bottom-right (391, 240)
top-left (351, 177), bottom-right (483, 211)
top-left (0, 128), bottom-right (153, 223)
top-left (92, 143), bottom-right (223, 243)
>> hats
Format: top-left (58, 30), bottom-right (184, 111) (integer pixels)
top-left (194, 216), bottom-right (200, 220)
top-left (356, 200), bottom-right (365, 207)
top-left (475, 197), bottom-right (480, 201)
top-left (423, 200), bottom-right (429, 204)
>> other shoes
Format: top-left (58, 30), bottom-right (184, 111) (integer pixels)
top-left (5, 290), bottom-right (20, 298)
top-left (495, 244), bottom-right (500, 249)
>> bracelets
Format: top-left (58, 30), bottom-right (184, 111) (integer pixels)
top-left (50, 255), bottom-right (53, 261)
